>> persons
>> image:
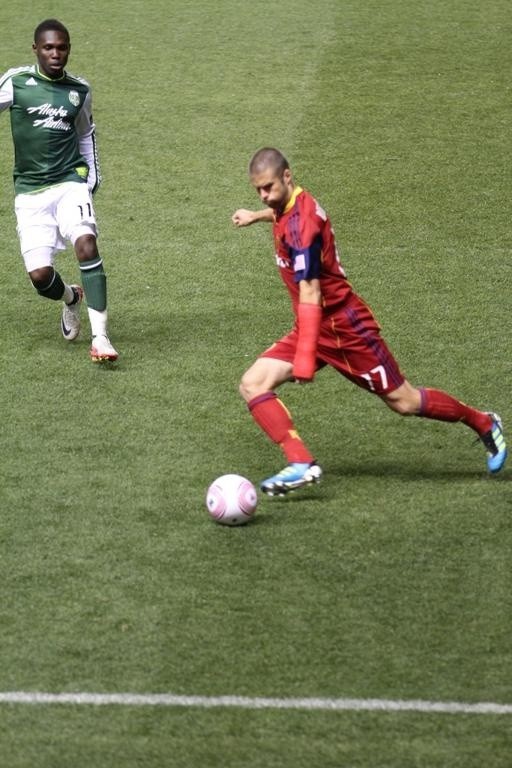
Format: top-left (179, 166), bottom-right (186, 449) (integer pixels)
top-left (0, 20), bottom-right (118, 363)
top-left (231, 148), bottom-right (508, 498)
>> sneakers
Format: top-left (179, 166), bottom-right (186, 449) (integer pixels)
top-left (260, 459), bottom-right (323, 497)
top-left (60, 284), bottom-right (83, 341)
top-left (470, 412), bottom-right (507, 472)
top-left (89, 335), bottom-right (118, 362)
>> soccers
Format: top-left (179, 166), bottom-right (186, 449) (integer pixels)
top-left (206, 473), bottom-right (258, 525)
